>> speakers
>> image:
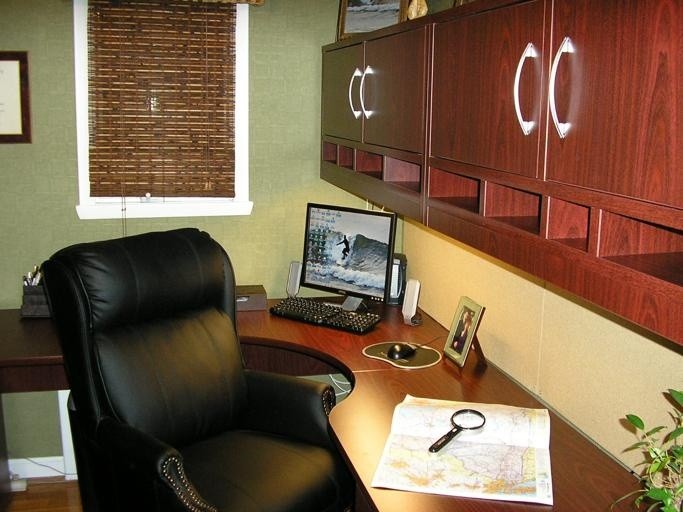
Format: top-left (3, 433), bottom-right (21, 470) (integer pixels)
top-left (401, 278), bottom-right (422, 326)
top-left (286, 261), bottom-right (302, 298)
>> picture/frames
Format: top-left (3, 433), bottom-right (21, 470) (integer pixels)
top-left (442, 297), bottom-right (488, 366)
top-left (336, 0), bottom-right (407, 41)
top-left (0, 50), bottom-right (30, 144)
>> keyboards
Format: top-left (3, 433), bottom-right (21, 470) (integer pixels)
top-left (269, 296), bottom-right (383, 336)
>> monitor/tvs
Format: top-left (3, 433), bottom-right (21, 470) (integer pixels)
top-left (300, 202), bottom-right (398, 312)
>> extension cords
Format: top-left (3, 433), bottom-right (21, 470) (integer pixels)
top-left (0, 478), bottom-right (28, 493)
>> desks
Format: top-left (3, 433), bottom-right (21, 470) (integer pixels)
top-left (0, 295), bottom-right (683, 511)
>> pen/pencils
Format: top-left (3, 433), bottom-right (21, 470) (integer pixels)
top-left (22, 264), bottom-right (42, 287)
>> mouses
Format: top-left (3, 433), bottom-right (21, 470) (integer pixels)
top-left (387, 343), bottom-right (417, 360)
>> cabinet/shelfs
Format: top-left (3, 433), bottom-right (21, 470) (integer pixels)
top-left (317, 16), bottom-right (426, 228)
top-left (427, 1), bottom-right (683, 355)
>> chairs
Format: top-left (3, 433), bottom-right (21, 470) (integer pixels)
top-left (36, 225), bottom-right (359, 511)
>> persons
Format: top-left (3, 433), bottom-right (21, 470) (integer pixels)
top-left (451, 312), bottom-right (471, 352)
top-left (335, 235), bottom-right (349, 260)
